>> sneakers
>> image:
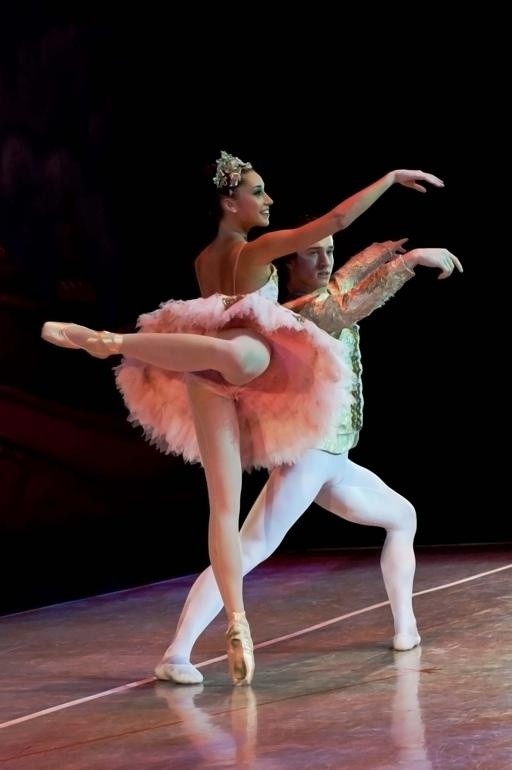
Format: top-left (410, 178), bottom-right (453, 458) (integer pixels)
top-left (40, 320), bottom-right (122, 360)
top-left (224, 611), bottom-right (256, 685)
top-left (154, 662), bottom-right (204, 684)
top-left (393, 634), bottom-right (422, 650)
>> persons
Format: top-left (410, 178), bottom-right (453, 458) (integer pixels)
top-left (40, 147), bottom-right (448, 689)
top-left (154, 232), bottom-right (467, 685)
